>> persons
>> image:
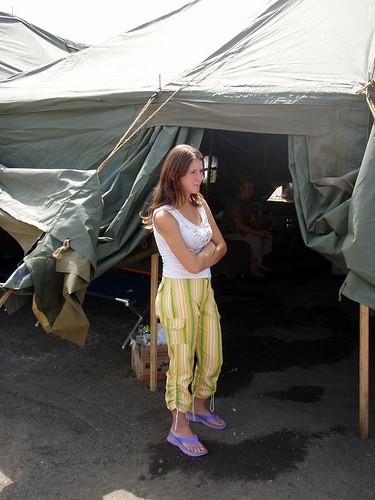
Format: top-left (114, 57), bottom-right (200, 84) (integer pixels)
top-left (222, 177), bottom-right (273, 280)
top-left (137, 144), bottom-right (227, 456)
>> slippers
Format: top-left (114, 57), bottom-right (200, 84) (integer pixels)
top-left (185, 407), bottom-right (227, 430)
top-left (167, 429), bottom-right (210, 457)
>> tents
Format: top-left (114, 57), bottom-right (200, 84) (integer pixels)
top-left (0, 10), bottom-right (90, 84)
top-left (0, 1), bottom-right (374, 440)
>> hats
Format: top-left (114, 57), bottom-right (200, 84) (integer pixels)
top-left (239, 176), bottom-right (257, 188)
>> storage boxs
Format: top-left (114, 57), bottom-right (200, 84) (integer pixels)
top-left (127, 329), bottom-right (171, 383)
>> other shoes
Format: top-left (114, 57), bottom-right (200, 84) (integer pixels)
top-left (251, 264), bottom-right (275, 275)
